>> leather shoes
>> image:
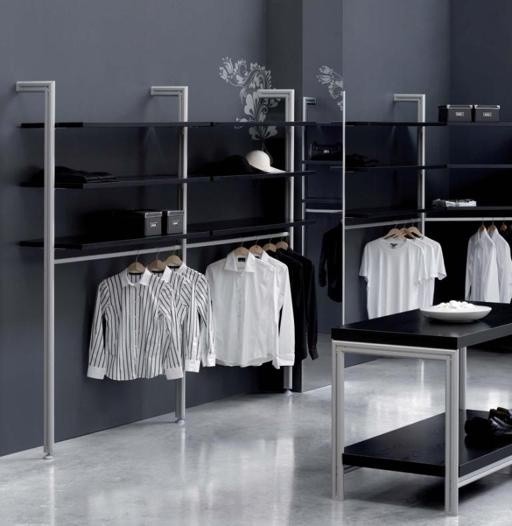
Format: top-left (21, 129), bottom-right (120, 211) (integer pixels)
top-left (490, 408), bottom-right (511, 423)
top-left (465, 416), bottom-right (512, 440)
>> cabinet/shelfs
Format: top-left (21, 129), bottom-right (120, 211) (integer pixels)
top-left (14, 80), bottom-right (297, 459)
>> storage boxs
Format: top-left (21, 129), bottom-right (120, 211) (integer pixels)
top-left (164, 210), bottom-right (185, 233)
top-left (437, 105), bottom-right (473, 123)
top-left (473, 105), bottom-right (500, 122)
top-left (125, 210), bottom-right (163, 237)
top-left (300, 91), bottom-right (511, 326)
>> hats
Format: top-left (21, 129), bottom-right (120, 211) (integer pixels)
top-left (244, 149), bottom-right (286, 173)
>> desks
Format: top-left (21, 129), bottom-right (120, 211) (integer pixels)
top-left (330, 301), bottom-right (511, 515)
